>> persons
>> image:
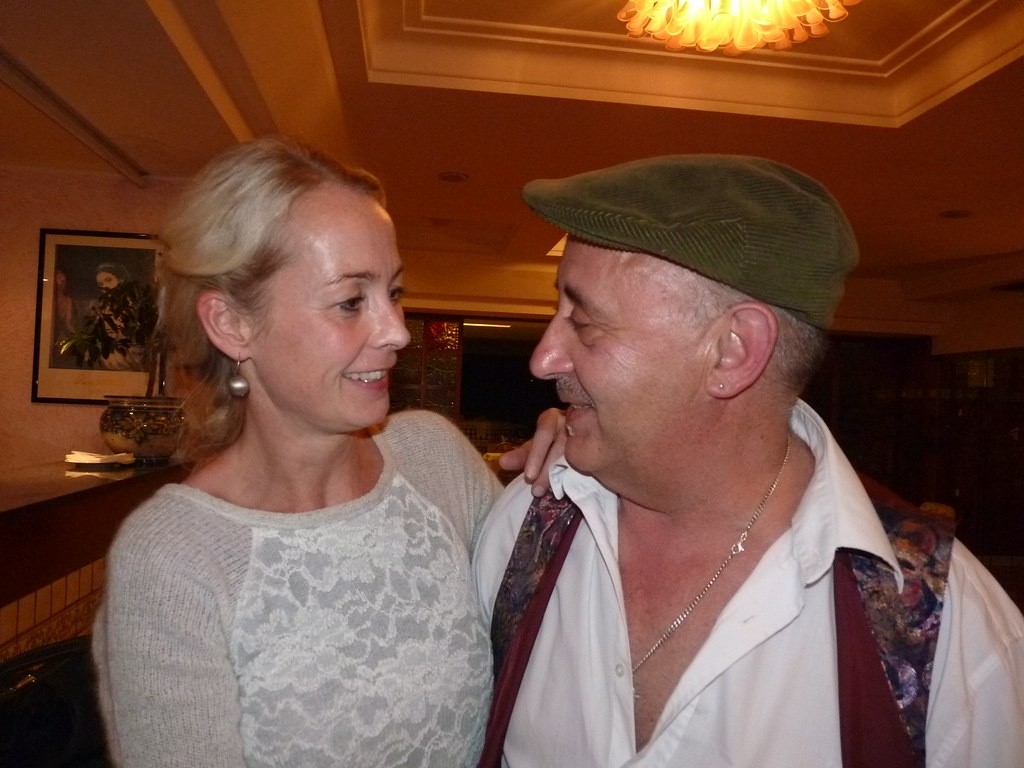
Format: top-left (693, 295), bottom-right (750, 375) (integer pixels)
top-left (474, 150), bottom-right (1023, 768)
top-left (90, 131), bottom-right (571, 768)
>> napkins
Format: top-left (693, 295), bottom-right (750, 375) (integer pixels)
top-left (64, 449), bottom-right (136, 465)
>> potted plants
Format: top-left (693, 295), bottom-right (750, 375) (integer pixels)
top-left (60, 273), bottom-right (188, 467)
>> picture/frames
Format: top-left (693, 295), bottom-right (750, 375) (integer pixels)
top-left (32, 227), bottom-right (169, 407)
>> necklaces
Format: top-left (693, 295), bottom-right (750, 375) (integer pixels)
top-left (627, 429), bottom-right (790, 708)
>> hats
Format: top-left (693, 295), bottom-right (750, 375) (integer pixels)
top-left (522, 152), bottom-right (861, 329)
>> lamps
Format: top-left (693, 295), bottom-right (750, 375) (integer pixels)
top-left (617, 0), bottom-right (858, 57)
